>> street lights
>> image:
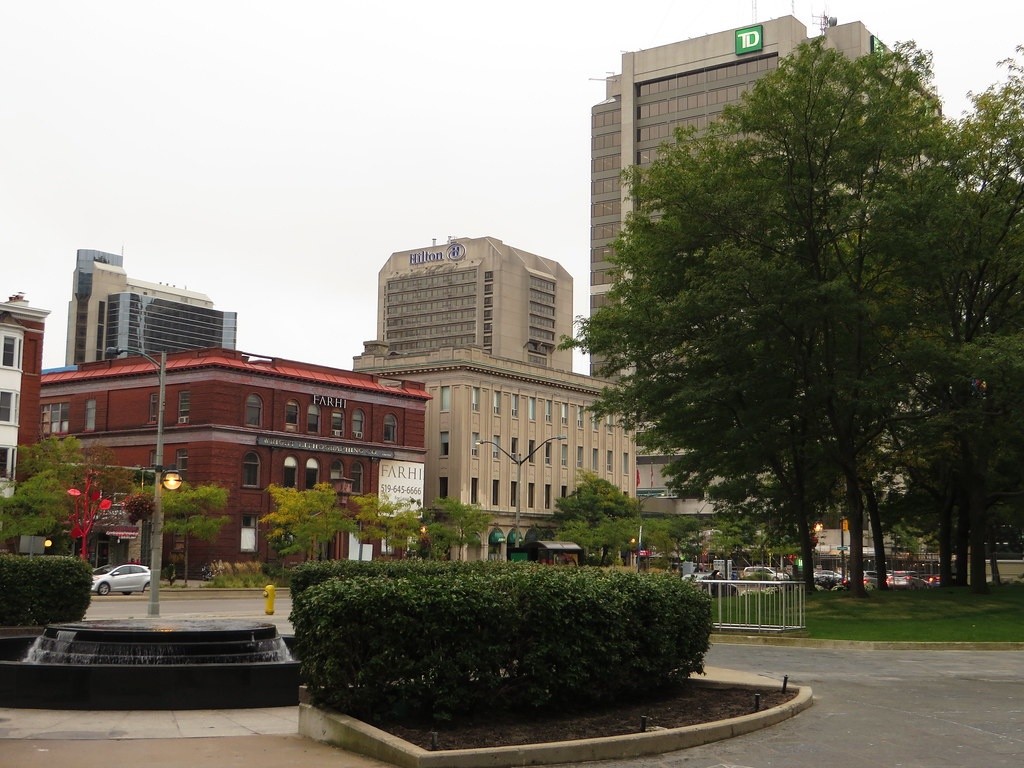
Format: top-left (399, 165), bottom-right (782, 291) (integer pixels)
top-left (474, 435), bottom-right (567, 547)
top-left (105, 344), bottom-right (183, 617)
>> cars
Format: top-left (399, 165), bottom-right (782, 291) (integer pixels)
top-left (90, 564), bottom-right (152, 595)
top-left (744, 567), bottom-right (790, 581)
top-left (864, 570), bottom-right (940, 592)
top-left (813, 570), bottom-right (842, 580)
top-left (681, 572), bottom-right (738, 597)
